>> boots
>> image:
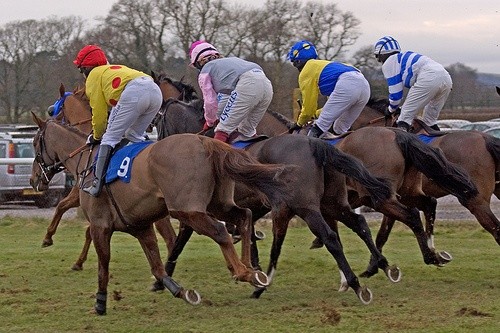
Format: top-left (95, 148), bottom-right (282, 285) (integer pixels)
top-left (84, 145), bottom-right (113, 198)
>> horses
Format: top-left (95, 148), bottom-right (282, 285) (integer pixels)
top-left (30, 70), bottom-right (500, 316)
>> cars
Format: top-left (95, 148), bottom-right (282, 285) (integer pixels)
top-left (0, 124), bottom-right (79, 208)
top-left (435, 117), bottom-right (500, 138)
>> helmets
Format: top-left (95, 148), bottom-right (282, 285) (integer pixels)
top-left (374, 36), bottom-right (401, 55)
top-left (288, 40), bottom-right (318, 60)
top-left (190, 40), bottom-right (218, 64)
top-left (74, 45), bottom-right (107, 67)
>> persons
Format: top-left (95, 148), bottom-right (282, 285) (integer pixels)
top-left (287, 40), bottom-right (371, 139)
top-left (73, 45), bottom-right (163, 197)
top-left (189, 41), bottom-right (273, 144)
top-left (373, 36), bottom-right (452, 132)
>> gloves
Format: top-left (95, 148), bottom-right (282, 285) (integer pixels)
top-left (385, 107), bottom-right (392, 117)
top-left (203, 122), bottom-right (210, 130)
top-left (289, 123), bottom-right (302, 134)
top-left (89, 136), bottom-right (101, 145)
